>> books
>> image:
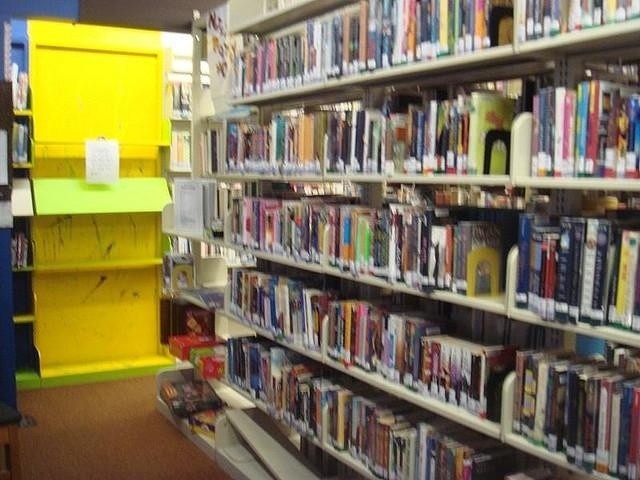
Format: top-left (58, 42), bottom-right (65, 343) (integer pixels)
top-left (307, 287), bottom-right (349, 361)
top-left (223, 335), bottom-right (267, 401)
top-left (170, 178), bottom-right (226, 240)
top-left (552, 344), bottom-right (638, 480)
top-left (9, 122), bottom-right (28, 163)
top-left (517, 0), bottom-right (639, 41)
top-left (411, 316), bottom-right (481, 408)
top-left (300, 12), bottom-right (359, 83)
top-left (372, 185), bottom-right (529, 296)
top-left (478, 439), bottom-right (550, 480)
top-left (307, 367), bottom-right (348, 451)
top-left (481, 342), bottom-right (549, 437)
top-left (530, 79), bottom-right (639, 178)
top-left (163, 259), bottom-right (229, 313)
top-left (410, 415), bottom-right (479, 480)
top-left (165, 332), bottom-right (223, 375)
top-left (233, 29), bottom-right (301, 95)
top-left (227, 267), bottom-right (269, 327)
top-left (348, 391), bottom-right (409, 480)
top-left (168, 238), bottom-right (226, 260)
top-left (350, 299), bottom-right (410, 380)
top-left (11, 62), bottom-right (28, 109)
top-left (290, 192), bottom-right (370, 273)
top-left (272, 90), bottom-right (521, 177)
top-left (422, 1), bottom-right (512, 57)
top-left (228, 179), bottom-right (292, 251)
top-left (9, 231), bottom-right (30, 270)
top-left (529, 190), bottom-right (639, 330)
top-left (159, 375), bottom-right (221, 437)
top-left (170, 130), bottom-right (191, 172)
top-left (358, 0), bottom-right (421, 71)
top-left (226, 119), bottom-right (273, 174)
top-left (168, 80), bottom-right (210, 117)
top-left (266, 346), bottom-right (306, 423)
top-left (269, 276), bottom-right (308, 344)
top-left (195, 123), bottom-right (225, 174)
